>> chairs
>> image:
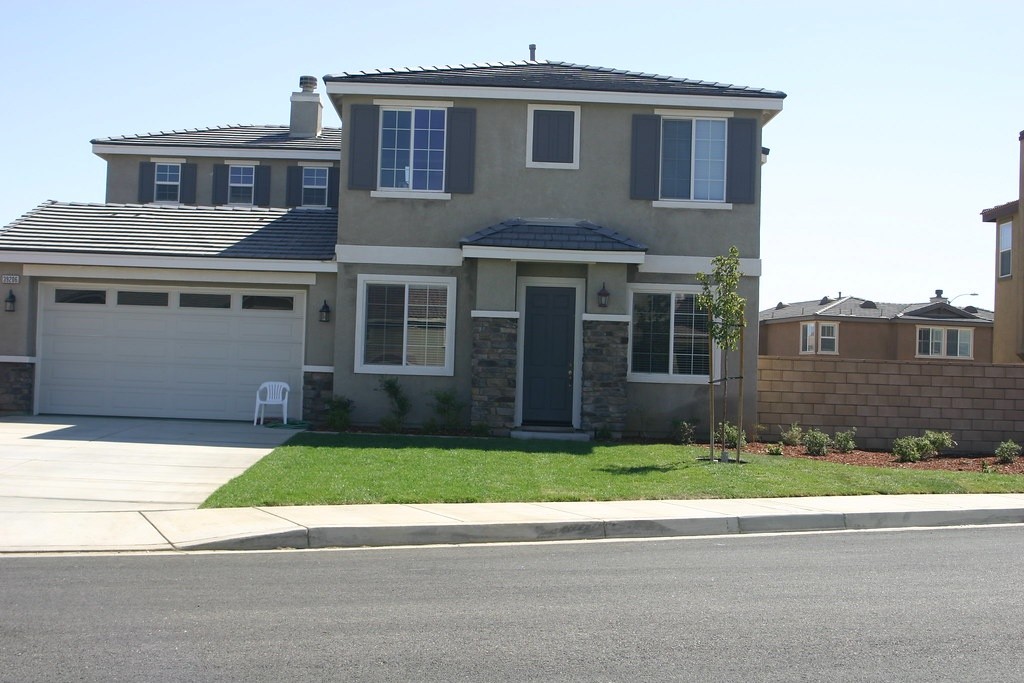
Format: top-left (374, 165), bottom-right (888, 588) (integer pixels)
top-left (254, 382), bottom-right (290, 426)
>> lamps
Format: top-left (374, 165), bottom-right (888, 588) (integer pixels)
top-left (319, 300), bottom-right (330, 322)
top-left (597, 282), bottom-right (609, 307)
top-left (5, 290), bottom-right (15, 312)
